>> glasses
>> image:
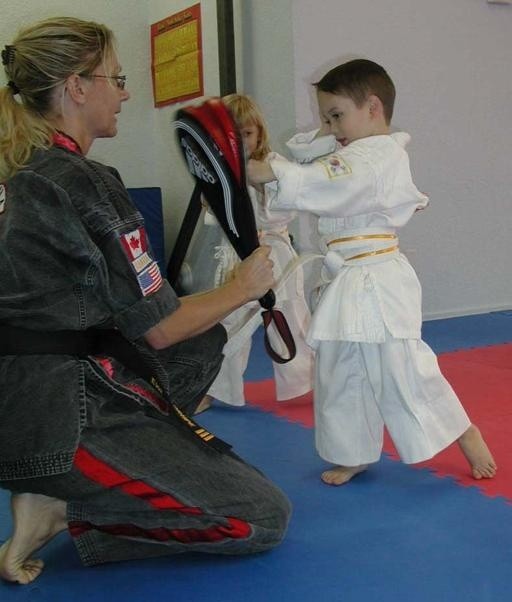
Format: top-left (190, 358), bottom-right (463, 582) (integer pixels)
top-left (75, 72), bottom-right (128, 92)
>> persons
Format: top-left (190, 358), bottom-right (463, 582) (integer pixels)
top-left (237, 56), bottom-right (500, 487)
top-left (181, 88), bottom-right (312, 418)
top-left (1, 16), bottom-right (284, 588)
